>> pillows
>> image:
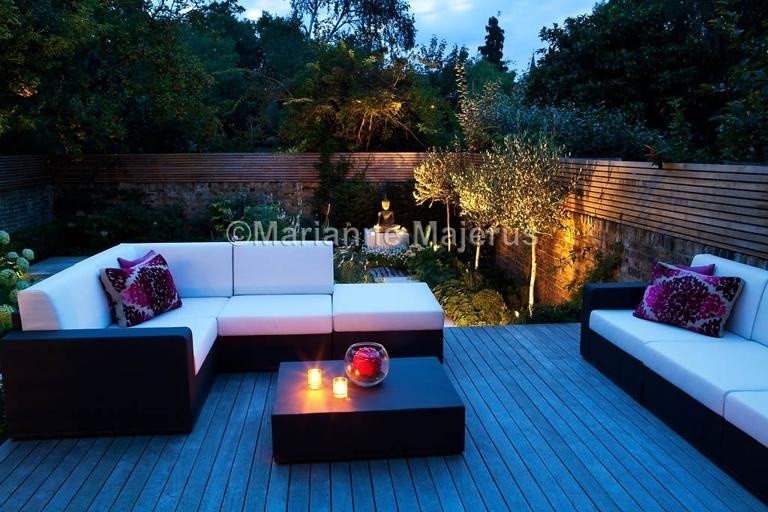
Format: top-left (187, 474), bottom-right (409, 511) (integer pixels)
top-left (675, 264), bottom-right (715, 276)
top-left (117, 250), bottom-right (157, 269)
top-left (633, 262), bottom-right (745, 338)
top-left (99, 252), bottom-right (182, 327)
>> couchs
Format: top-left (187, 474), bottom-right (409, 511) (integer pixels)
top-left (580, 253), bottom-right (768, 508)
top-left (0, 240), bottom-right (446, 441)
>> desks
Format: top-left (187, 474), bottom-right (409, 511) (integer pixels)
top-left (270, 356), bottom-right (465, 465)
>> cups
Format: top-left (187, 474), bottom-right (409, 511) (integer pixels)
top-left (332, 376), bottom-right (348, 399)
top-left (307, 367), bottom-right (323, 390)
